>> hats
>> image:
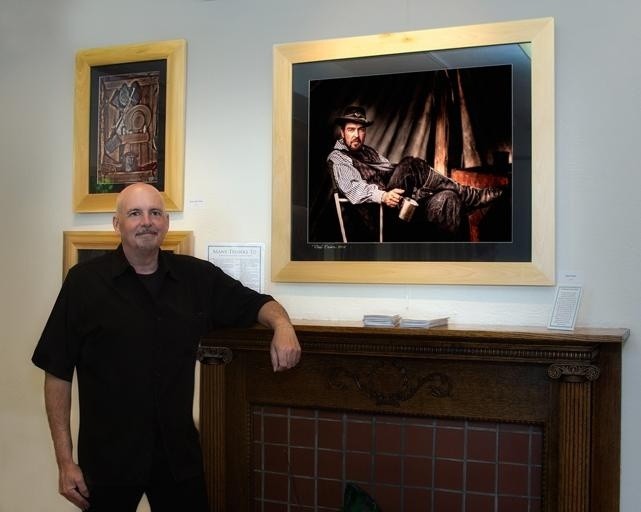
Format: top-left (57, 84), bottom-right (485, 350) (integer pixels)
top-left (334, 104), bottom-right (376, 129)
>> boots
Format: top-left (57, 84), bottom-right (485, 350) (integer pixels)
top-left (420, 166), bottom-right (506, 214)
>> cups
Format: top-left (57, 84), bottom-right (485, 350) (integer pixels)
top-left (398, 196), bottom-right (420, 222)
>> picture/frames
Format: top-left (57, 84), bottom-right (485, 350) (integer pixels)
top-left (63, 39), bottom-right (193, 289)
top-left (270, 15), bottom-right (556, 286)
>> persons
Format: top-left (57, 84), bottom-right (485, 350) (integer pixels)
top-left (26, 180), bottom-right (305, 512)
top-left (324, 101), bottom-right (510, 241)
